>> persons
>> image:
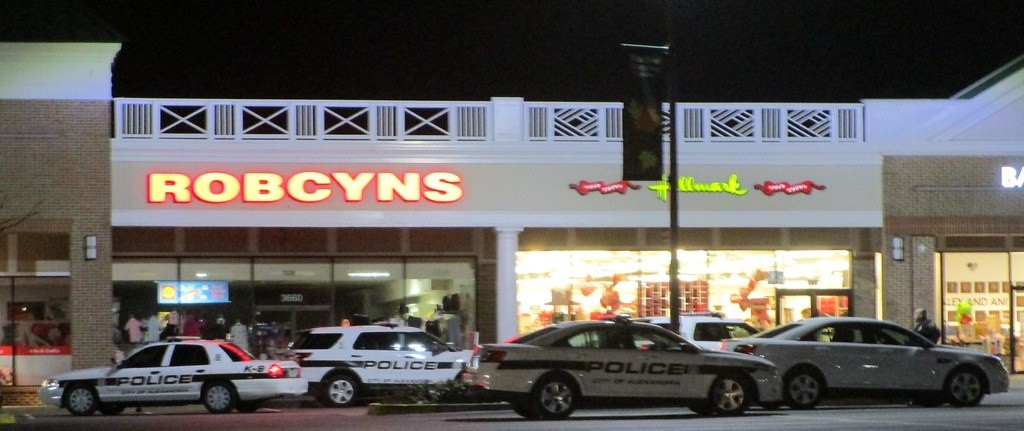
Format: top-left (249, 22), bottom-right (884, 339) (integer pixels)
top-left (744, 317), bottom-right (772, 332)
top-left (912, 308), bottom-right (940, 345)
top-left (800, 308), bottom-right (811, 319)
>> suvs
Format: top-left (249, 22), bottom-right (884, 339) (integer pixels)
top-left (281, 319), bottom-right (474, 409)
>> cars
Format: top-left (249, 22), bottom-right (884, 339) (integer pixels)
top-left (568, 312), bottom-right (760, 353)
top-left (38, 336), bottom-right (308, 418)
top-left (463, 314), bottom-right (784, 422)
top-left (718, 316), bottom-right (1010, 410)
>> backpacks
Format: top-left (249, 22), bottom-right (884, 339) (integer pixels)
top-left (922, 320), bottom-right (941, 344)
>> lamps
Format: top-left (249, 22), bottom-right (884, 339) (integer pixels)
top-left (84, 233), bottom-right (98, 261)
top-left (892, 237), bottom-right (903, 262)
